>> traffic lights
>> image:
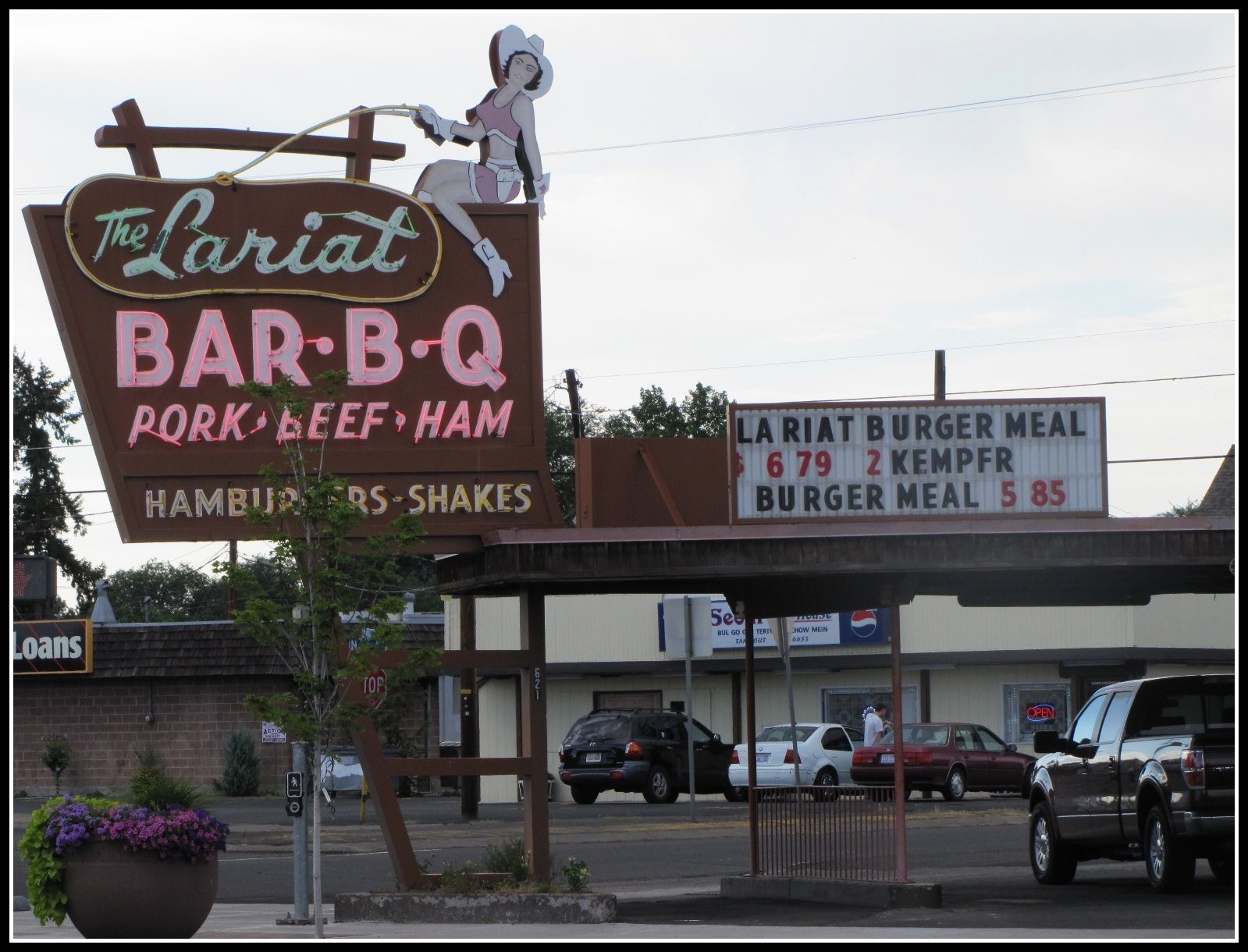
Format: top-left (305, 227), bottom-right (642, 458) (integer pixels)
top-left (14, 554), bottom-right (58, 604)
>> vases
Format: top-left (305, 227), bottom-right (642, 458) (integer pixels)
top-left (59, 838), bottom-right (219, 938)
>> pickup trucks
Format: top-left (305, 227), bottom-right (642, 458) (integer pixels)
top-left (1021, 674), bottom-right (1234, 891)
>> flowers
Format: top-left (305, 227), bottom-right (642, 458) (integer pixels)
top-left (17, 792), bottom-right (232, 930)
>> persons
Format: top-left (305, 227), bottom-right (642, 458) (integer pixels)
top-left (863, 702), bottom-right (887, 797)
top-left (410, 22), bottom-right (556, 298)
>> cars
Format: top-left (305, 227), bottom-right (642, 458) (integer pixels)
top-left (850, 721), bottom-right (1040, 800)
top-left (727, 721), bottom-right (865, 802)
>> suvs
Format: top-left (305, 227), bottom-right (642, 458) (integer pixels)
top-left (557, 706), bottom-right (748, 804)
